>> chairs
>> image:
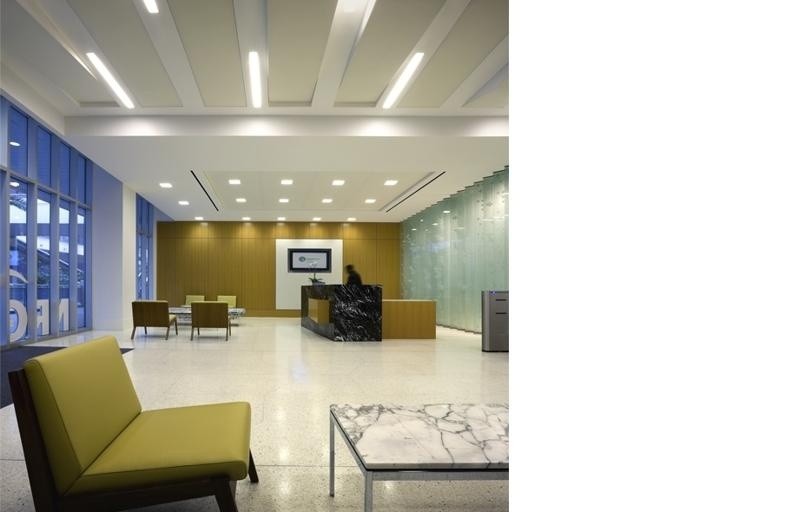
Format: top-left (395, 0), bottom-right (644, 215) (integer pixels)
top-left (131, 295), bottom-right (236, 340)
top-left (7, 334), bottom-right (259, 512)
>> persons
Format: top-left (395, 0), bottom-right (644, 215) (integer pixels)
top-left (344, 264), bottom-right (362, 284)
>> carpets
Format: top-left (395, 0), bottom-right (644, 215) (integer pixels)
top-left (0, 345), bottom-right (135, 410)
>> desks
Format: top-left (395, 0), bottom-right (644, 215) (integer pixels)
top-left (168, 307), bottom-right (246, 326)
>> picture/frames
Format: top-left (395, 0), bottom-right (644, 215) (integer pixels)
top-left (287, 248), bottom-right (332, 273)
